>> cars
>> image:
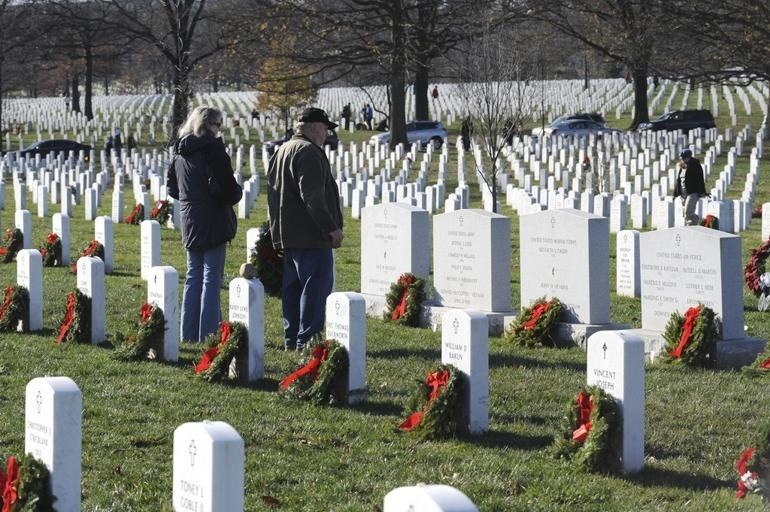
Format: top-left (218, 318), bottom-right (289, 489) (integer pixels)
top-left (531, 111), bottom-right (605, 137)
top-left (636, 109), bottom-right (715, 137)
top-left (2, 139), bottom-right (92, 163)
top-left (263, 127), bottom-right (338, 156)
top-left (538, 119), bottom-right (622, 142)
top-left (369, 120), bottom-right (448, 150)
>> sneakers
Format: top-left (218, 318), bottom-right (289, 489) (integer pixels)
top-left (286, 338), bottom-right (307, 349)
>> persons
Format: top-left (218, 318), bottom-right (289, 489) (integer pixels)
top-left (267, 105), bottom-right (346, 352)
top-left (365, 103), bottom-right (374, 130)
top-left (503, 117), bottom-right (523, 145)
top-left (167, 105), bottom-right (243, 342)
top-left (432, 86), bottom-right (439, 99)
top-left (342, 103), bottom-right (352, 130)
top-left (460, 116), bottom-right (474, 151)
top-left (674, 149), bottom-right (708, 226)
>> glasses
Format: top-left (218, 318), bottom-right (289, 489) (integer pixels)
top-left (210, 122), bottom-right (221, 127)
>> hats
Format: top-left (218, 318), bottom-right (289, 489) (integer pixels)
top-left (299, 108), bottom-right (339, 130)
top-left (681, 150), bottom-right (692, 157)
